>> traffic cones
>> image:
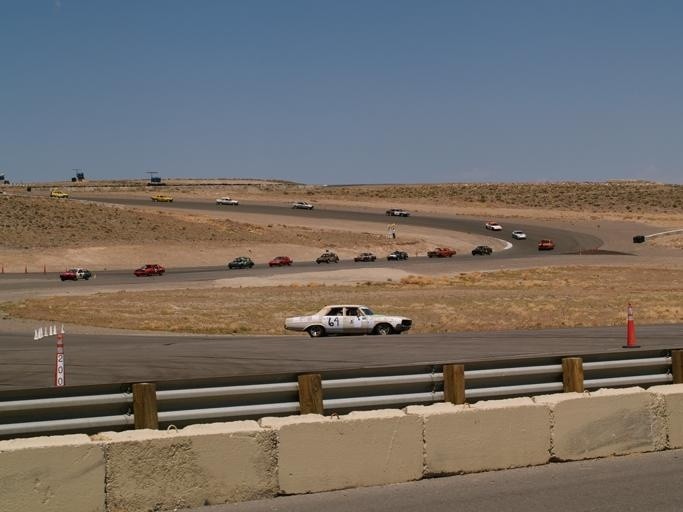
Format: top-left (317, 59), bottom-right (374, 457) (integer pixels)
top-left (622, 301), bottom-right (640, 348)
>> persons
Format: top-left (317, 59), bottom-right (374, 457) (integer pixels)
top-left (392, 227), bottom-right (397, 239)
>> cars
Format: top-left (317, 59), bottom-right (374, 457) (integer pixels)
top-left (284, 304), bottom-right (413, 337)
top-left (51, 190), bottom-right (69, 198)
top-left (386, 208), bottom-right (409, 216)
top-left (151, 195), bottom-right (174, 202)
top-left (133, 262), bottom-right (166, 277)
top-left (216, 197), bottom-right (238, 205)
top-left (60, 267), bottom-right (91, 281)
top-left (292, 200), bottom-right (313, 209)
